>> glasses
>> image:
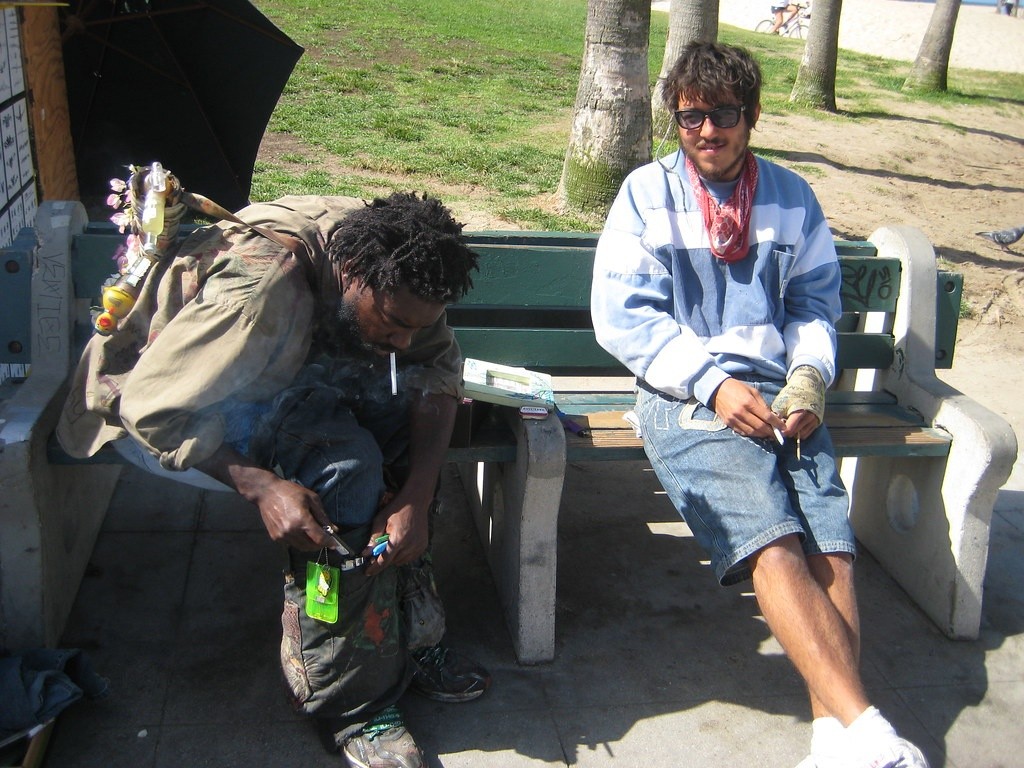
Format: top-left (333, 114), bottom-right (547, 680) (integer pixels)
top-left (673, 105), bottom-right (749, 129)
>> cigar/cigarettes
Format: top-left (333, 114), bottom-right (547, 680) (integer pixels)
top-left (391, 353), bottom-right (397, 395)
top-left (772, 424), bottom-right (784, 445)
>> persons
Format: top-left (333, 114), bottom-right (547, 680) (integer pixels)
top-left (591, 40), bottom-right (928, 768)
top-left (769, 0), bottom-right (797, 34)
top-left (1005, 0), bottom-right (1014, 16)
top-left (46, 195), bottom-right (486, 768)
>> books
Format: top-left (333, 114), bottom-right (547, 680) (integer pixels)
top-left (463, 358), bottom-right (554, 413)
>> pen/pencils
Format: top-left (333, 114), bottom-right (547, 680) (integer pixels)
top-left (375, 535), bottom-right (388, 544)
top-left (796, 430), bottom-right (800, 462)
top-left (373, 541), bottom-right (388, 556)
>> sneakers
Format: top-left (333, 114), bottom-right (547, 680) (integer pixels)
top-left (407, 646), bottom-right (493, 702)
top-left (338, 702), bottom-right (429, 768)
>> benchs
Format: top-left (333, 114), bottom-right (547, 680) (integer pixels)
top-left (0, 221), bottom-right (1017, 666)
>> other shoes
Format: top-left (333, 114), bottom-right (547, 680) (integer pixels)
top-left (798, 734), bottom-right (931, 768)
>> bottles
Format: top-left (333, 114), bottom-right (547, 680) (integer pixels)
top-left (94, 255), bottom-right (158, 337)
top-left (142, 188), bottom-right (166, 257)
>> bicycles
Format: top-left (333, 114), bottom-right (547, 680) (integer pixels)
top-left (753, 4), bottom-right (810, 40)
